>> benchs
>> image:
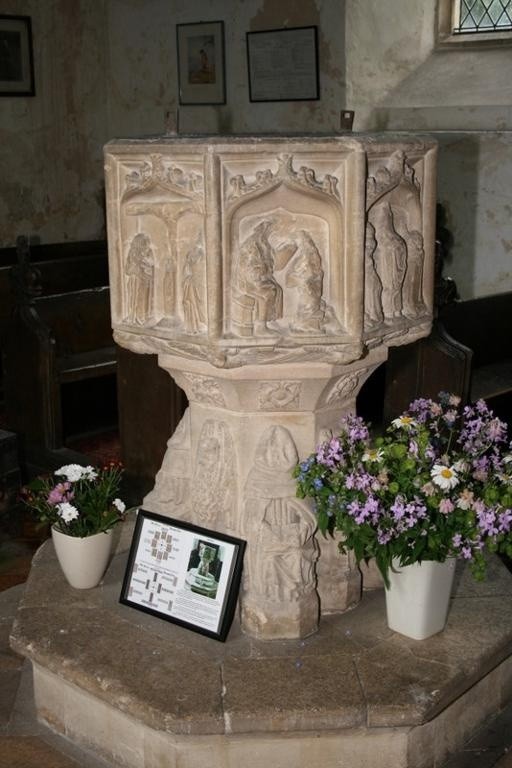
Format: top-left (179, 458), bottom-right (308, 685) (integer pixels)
top-left (17, 285), bottom-right (117, 450)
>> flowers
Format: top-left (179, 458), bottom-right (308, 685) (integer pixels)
top-left (18, 463), bottom-right (128, 541)
top-left (287, 390), bottom-right (512, 589)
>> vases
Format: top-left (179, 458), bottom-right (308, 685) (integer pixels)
top-left (385, 554), bottom-right (456, 642)
top-left (50, 523), bottom-right (121, 589)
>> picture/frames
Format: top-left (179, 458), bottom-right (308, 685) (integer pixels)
top-left (174, 19), bottom-right (320, 105)
top-left (0, 14), bottom-right (36, 96)
top-left (117, 508), bottom-right (247, 643)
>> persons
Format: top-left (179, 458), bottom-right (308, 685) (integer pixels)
top-left (199, 48), bottom-right (209, 68)
top-left (120, 198), bottom-right (430, 335)
